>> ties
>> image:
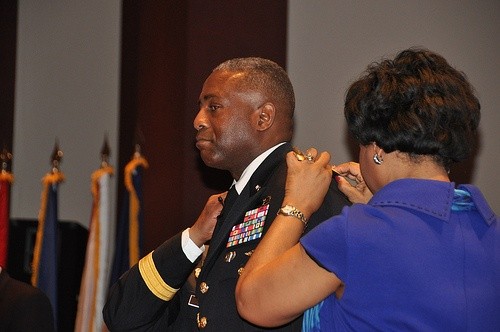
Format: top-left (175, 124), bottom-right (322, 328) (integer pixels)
top-left (206, 184), bottom-right (240, 266)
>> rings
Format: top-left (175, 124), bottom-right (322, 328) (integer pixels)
top-left (305, 154), bottom-right (316, 161)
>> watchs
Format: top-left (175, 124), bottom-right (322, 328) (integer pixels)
top-left (276, 205), bottom-right (309, 230)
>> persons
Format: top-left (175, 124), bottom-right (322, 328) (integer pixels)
top-left (102, 190), bottom-right (229, 332)
top-left (192, 57), bottom-right (352, 332)
top-left (235, 46), bottom-right (500, 332)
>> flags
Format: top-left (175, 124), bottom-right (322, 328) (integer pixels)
top-left (109, 156), bottom-right (148, 285)
top-left (73, 166), bottom-right (116, 332)
top-left (0, 171), bottom-right (14, 270)
top-left (31, 173), bottom-right (65, 332)
